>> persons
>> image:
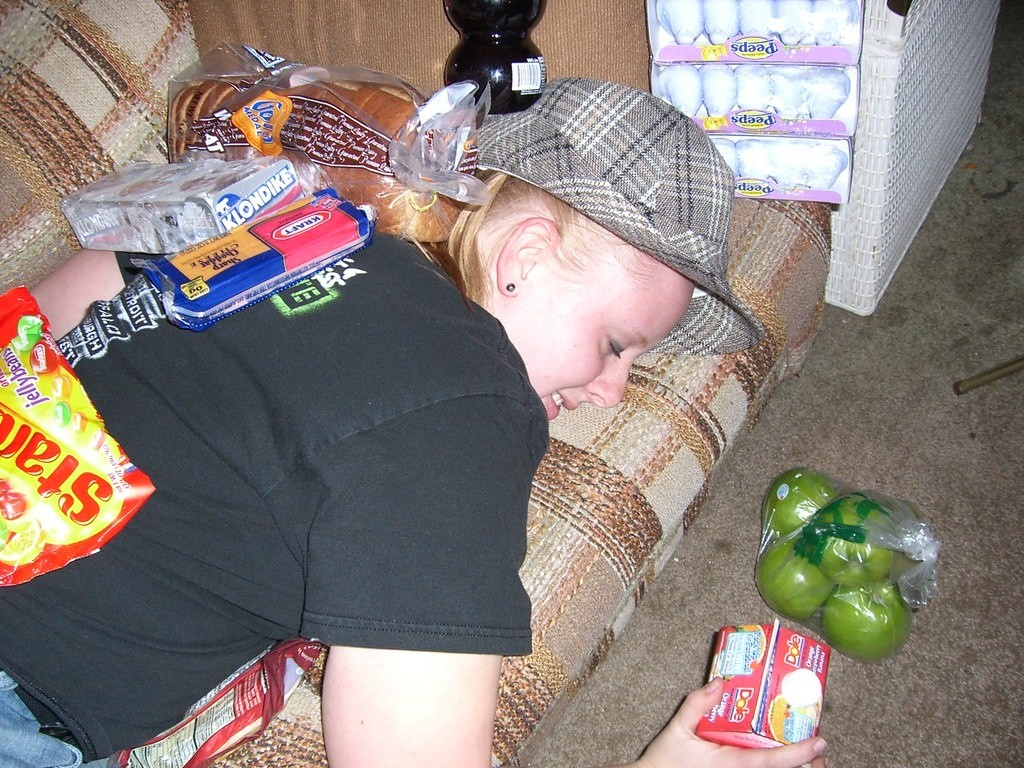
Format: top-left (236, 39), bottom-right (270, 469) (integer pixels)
top-left (0, 75), bottom-right (829, 768)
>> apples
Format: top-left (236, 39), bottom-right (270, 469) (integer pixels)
top-left (759, 469), bottom-right (910, 661)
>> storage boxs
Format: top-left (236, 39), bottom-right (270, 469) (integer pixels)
top-left (696, 618), bottom-right (831, 768)
top-left (645, 0), bottom-right (866, 204)
top-left (61, 161), bottom-right (302, 254)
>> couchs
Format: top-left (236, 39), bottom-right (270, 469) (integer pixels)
top-left (0, 0), bottom-right (833, 768)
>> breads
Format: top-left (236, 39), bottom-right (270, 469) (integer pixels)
top-left (169, 73), bottom-right (460, 242)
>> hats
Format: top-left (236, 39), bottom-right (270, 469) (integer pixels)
top-left (477, 77), bottom-right (766, 357)
